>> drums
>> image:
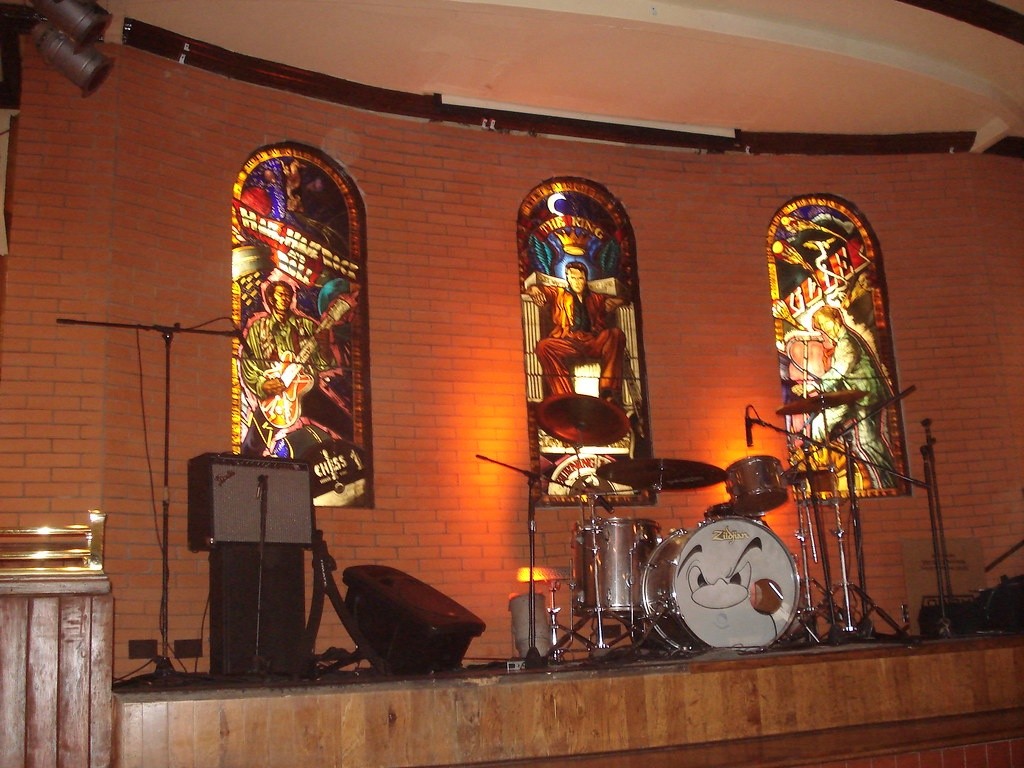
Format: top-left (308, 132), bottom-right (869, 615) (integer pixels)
top-left (640, 518), bottom-right (802, 652)
top-left (567, 518), bottom-right (662, 616)
top-left (723, 454), bottom-right (789, 518)
top-left (703, 499), bottom-right (768, 525)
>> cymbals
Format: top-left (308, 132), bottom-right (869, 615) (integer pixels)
top-left (776, 389), bottom-right (868, 414)
top-left (595, 455), bottom-right (729, 490)
top-left (536, 393), bottom-right (630, 447)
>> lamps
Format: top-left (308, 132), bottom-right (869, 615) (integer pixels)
top-left (509, 593), bottom-right (553, 660)
top-left (29, 0), bottom-right (114, 48)
top-left (27, 19), bottom-right (117, 99)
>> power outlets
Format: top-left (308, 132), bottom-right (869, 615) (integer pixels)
top-left (127, 639), bottom-right (159, 659)
top-left (173, 638), bottom-right (204, 659)
top-left (601, 623), bottom-right (622, 639)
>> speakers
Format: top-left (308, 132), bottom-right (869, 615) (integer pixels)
top-left (342, 565), bottom-right (487, 677)
top-left (209, 542), bottom-right (307, 688)
top-left (186, 453), bottom-right (316, 554)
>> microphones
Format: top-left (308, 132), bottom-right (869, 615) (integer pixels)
top-left (745, 410), bottom-right (753, 447)
top-left (597, 495), bottom-right (615, 515)
top-left (256, 474), bottom-right (264, 498)
top-left (229, 318), bottom-right (255, 358)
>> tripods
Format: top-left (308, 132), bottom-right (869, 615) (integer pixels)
top-left (55, 318), bottom-right (237, 689)
top-left (543, 490), bottom-right (682, 667)
top-left (751, 384), bottom-right (921, 649)
